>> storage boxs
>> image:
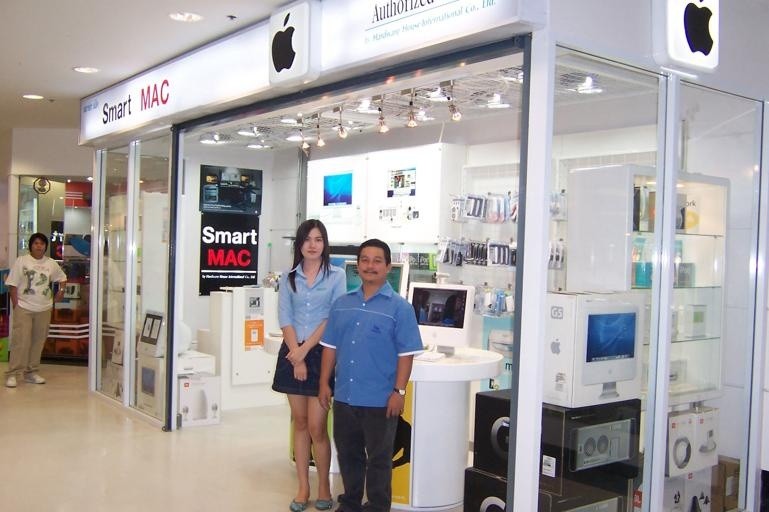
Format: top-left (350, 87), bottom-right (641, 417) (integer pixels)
top-left (472, 388), bottom-right (642, 498)
top-left (40, 276), bottom-right (92, 360)
top-left (665, 406), bottom-right (720, 479)
top-left (542, 292), bottom-right (646, 410)
top-left (665, 467), bottom-right (713, 512)
top-left (465, 465), bottom-right (626, 511)
top-left (101, 334), bottom-right (225, 429)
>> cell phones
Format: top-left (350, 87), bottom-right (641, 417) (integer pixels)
top-left (140, 365), bottom-right (156, 409)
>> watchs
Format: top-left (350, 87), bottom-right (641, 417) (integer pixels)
top-left (393, 387), bottom-right (406, 396)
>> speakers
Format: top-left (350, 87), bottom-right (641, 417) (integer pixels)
top-left (563, 496), bottom-right (623, 512)
top-left (569, 418), bottom-right (636, 472)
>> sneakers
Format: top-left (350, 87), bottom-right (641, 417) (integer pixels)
top-left (6, 377), bottom-right (17, 388)
top-left (316, 498), bottom-right (334, 509)
top-left (291, 497), bottom-right (307, 511)
top-left (24, 374), bottom-right (44, 384)
top-left (337, 499), bottom-right (390, 512)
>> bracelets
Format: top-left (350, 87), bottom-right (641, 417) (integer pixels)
top-left (60, 288), bottom-right (65, 292)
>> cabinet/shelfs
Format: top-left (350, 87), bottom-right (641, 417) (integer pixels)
top-left (105, 190), bottom-right (165, 334)
top-left (568, 164), bottom-right (732, 409)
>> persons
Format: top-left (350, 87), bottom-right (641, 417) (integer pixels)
top-left (441, 295), bottom-right (463, 327)
top-left (4, 232), bottom-right (69, 389)
top-left (271, 218), bottom-right (349, 512)
top-left (317, 238), bottom-right (426, 511)
top-left (419, 290), bottom-right (431, 322)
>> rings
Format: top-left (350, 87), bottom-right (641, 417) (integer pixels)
top-left (293, 361), bottom-right (297, 365)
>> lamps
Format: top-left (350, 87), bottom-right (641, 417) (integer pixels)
top-left (198, 78), bottom-right (462, 151)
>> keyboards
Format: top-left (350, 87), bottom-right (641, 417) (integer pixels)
top-left (412, 350), bottom-right (444, 361)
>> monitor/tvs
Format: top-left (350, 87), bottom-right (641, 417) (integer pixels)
top-left (407, 282), bottom-right (475, 357)
top-left (582, 305), bottom-right (638, 399)
top-left (343, 260), bottom-right (409, 299)
top-left (322, 170), bottom-right (355, 208)
